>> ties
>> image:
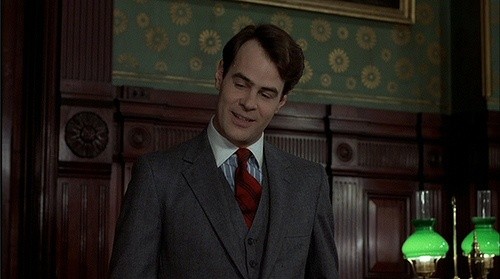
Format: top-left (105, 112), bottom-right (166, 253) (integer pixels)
top-left (234, 148), bottom-right (261, 230)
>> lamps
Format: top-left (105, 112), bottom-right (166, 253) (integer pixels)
top-left (460, 190), bottom-right (500, 279)
top-left (400, 189), bottom-right (449, 279)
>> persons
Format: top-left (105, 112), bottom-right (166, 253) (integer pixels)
top-left (106, 24), bottom-right (339, 278)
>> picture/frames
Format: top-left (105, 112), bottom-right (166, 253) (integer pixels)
top-left (247, 0), bottom-right (417, 26)
